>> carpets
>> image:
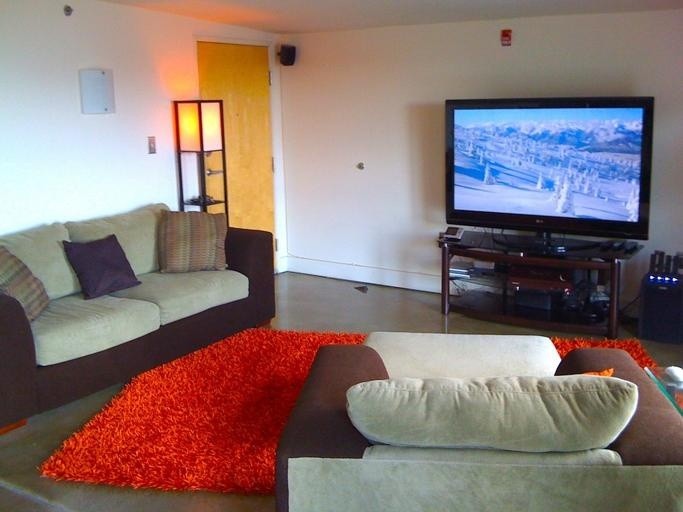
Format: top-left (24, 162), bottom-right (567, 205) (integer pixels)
top-left (34, 327), bottom-right (683, 500)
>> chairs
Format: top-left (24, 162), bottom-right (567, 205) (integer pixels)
top-left (275, 332), bottom-right (683, 512)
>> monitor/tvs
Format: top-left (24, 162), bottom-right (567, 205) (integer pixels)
top-left (444, 94), bottom-right (655, 250)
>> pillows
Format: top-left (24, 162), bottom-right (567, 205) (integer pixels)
top-left (0, 221), bottom-right (82, 301)
top-left (0, 244), bottom-right (50, 322)
top-left (345, 373), bottom-right (639, 452)
top-left (63, 234), bottom-right (142, 300)
top-left (63, 203), bottom-right (171, 276)
top-left (159, 208), bottom-right (227, 273)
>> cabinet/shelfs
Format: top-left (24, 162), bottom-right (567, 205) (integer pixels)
top-left (437, 231), bottom-right (646, 339)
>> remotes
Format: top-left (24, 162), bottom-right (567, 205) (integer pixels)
top-left (600, 239), bottom-right (638, 253)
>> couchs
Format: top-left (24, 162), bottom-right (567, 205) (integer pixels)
top-left (0, 227), bottom-right (276, 435)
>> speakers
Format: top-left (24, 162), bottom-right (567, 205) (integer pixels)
top-left (279, 44), bottom-right (296, 65)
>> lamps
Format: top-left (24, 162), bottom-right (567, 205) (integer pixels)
top-left (277, 44), bottom-right (296, 66)
